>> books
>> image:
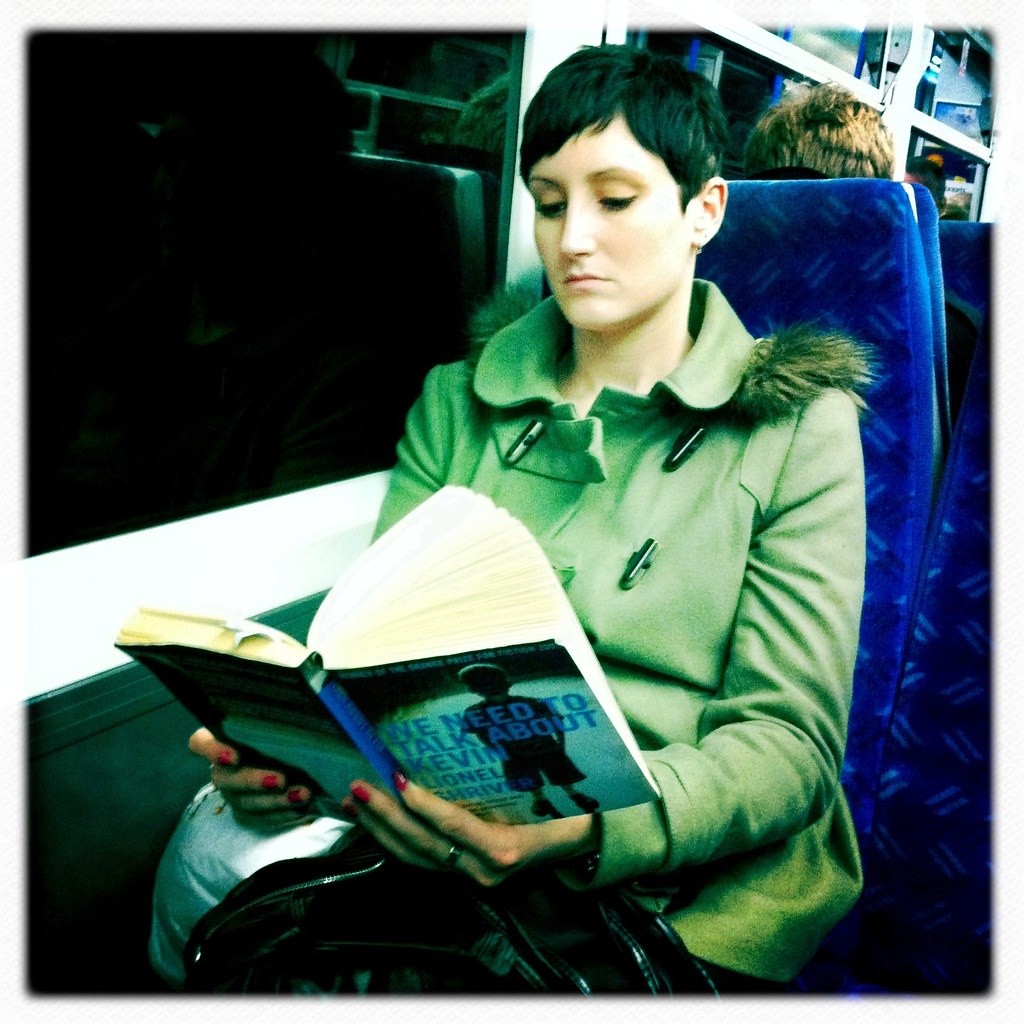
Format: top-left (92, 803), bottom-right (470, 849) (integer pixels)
top-left (114, 486), bottom-right (663, 828)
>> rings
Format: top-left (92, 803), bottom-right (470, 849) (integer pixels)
top-left (442, 842), bottom-right (466, 872)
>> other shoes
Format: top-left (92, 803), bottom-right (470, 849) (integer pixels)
top-left (569, 791), bottom-right (600, 814)
top-left (531, 798), bottom-right (565, 819)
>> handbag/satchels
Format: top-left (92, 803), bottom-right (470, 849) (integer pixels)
top-left (179, 823), bottom-right (721, 997)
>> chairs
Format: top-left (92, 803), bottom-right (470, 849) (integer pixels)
top-left (465, 178), bottom-right (957, 996)
top-left (866, 222), bottom-right (991, 998)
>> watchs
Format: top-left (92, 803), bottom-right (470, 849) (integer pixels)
top-left (568, 852), bottom-right (599, 883)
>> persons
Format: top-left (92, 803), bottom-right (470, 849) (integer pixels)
top-left (186, 46), bottom-right (867, 993)
top-left (904, 156), bottom-right (966, 220)
top-left (456, 660), bottom-right (600, 817)
top-left (741, 84), bottom-right (894, 178)
